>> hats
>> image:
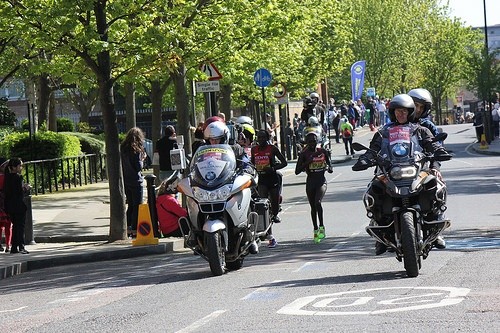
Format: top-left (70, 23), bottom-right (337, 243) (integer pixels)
top-left (305, 128), bottom-right (319, 138)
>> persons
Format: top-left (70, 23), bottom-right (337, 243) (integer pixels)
top-left (368, 87), bottom-right (445, 256)
top-left (443, 117), bottom-right (449, 125)
top-left (0, 156), bottom-right (33, 254)
top-left (119, 127), bottom-right (152, 240)
top-left (354, 94), bottom-right (449, 219)
top-left (285, 93), bottom-right (435, 160)
top-left (156, 112), bottom-right (287, 255)
top-left (455, 99), bottom-right (500, 143)
top-left (294, 133), bottom-right (333, 245)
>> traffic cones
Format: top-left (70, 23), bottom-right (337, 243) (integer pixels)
top-left (131, 204), bottom-right (159, 246)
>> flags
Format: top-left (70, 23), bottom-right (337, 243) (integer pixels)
top-left (351, 61), bottom-right (365, 102)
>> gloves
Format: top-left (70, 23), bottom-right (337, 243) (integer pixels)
top-left (434, 149), bottom-right (451, 161)
top-left (353, 159), bottom-right (369, 171)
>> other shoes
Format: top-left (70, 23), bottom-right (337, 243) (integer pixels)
top-left (0, 247), bottom-right (4, 251)
top-left (10, 248), bottom-right (20, 254)
top-left (5, 249), bottom-right (10, 253)
top-left (273, 215), bottom-right (281, 223)
top-left (434, 236), bottom-right (446, 249)
top-left (268, 238), bottom-right (277, 248)
top-left (19, 249), bottom-right (29, 254)
top-left (375, 241), bottom-right (387, 255)
top-left (248, 240), bottom-right (258, 254)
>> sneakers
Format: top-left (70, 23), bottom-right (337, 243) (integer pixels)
top-left (319, 226), bottom-right (325, 239)
top-left (314, 229), bottom-right (320, 244)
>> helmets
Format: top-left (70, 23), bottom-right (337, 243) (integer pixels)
top-left (406, 89), bottom-right (433, 114)
top-left (388, 94), bottom-right (416, 123)
top-left (203, 121), bottom-right (229, 139)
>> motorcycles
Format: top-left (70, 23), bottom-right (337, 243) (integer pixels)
top-left (173, 144), bottom-right (275, 276)
top-left (352, 133), bottom-right (455, 278)
